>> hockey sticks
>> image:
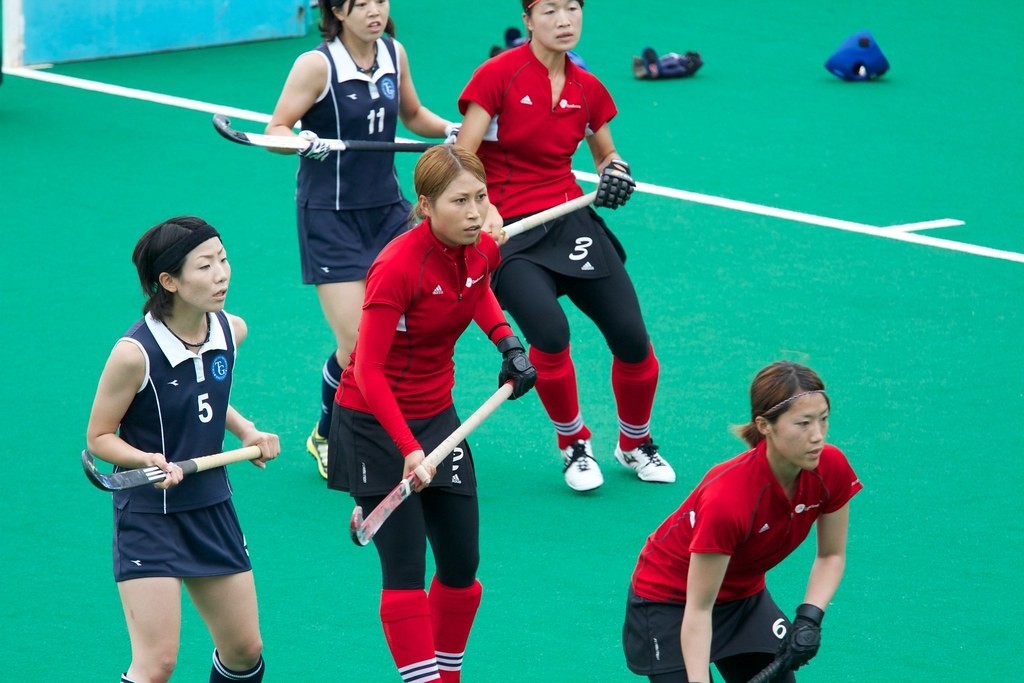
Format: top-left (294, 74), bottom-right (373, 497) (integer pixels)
top-left (350, 384), bottom-right (516, 546)
top-left (492, 190), bottom-right (602, 246)
top-left (211, 113), bottom-right (442, 152)
top-left (82, 444), bottom-right (272, 492)
top-left (747, 654), bottom-right (789, 683)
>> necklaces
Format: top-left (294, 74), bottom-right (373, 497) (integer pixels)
top-left (356, 63), bottom-right (376, 73)
top-left (160, 312), bottom-right (212, 346)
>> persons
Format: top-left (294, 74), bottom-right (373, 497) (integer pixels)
top-left (623, 361), bottom-right (864, 683)
top-left (87, 216), bottom-right (281, 683)
top-left (325, 143), bottom-right (537, 683)
top-left (455, 0), bottom-right (678, 493)
top-left (265, 0), bottom-right (463, 479)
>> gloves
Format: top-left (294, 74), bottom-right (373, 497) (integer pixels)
top-left (593, 158), bottom-right (636, 209)
top-left (297, 130), bottom-right (330, 162)
top-left (497, 336), bottom-right (538, 400)
top-left (443, 123), bottom-right (462, 144)
top-left (775, 603), bottom-right (825, 673)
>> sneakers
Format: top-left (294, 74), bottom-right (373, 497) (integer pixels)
top-left (614, 438), bottom-right (676, 483)
top-left (305, 421), bottom-right (328, 481)
top-left (560, 438), bottom-right (604, 492)
